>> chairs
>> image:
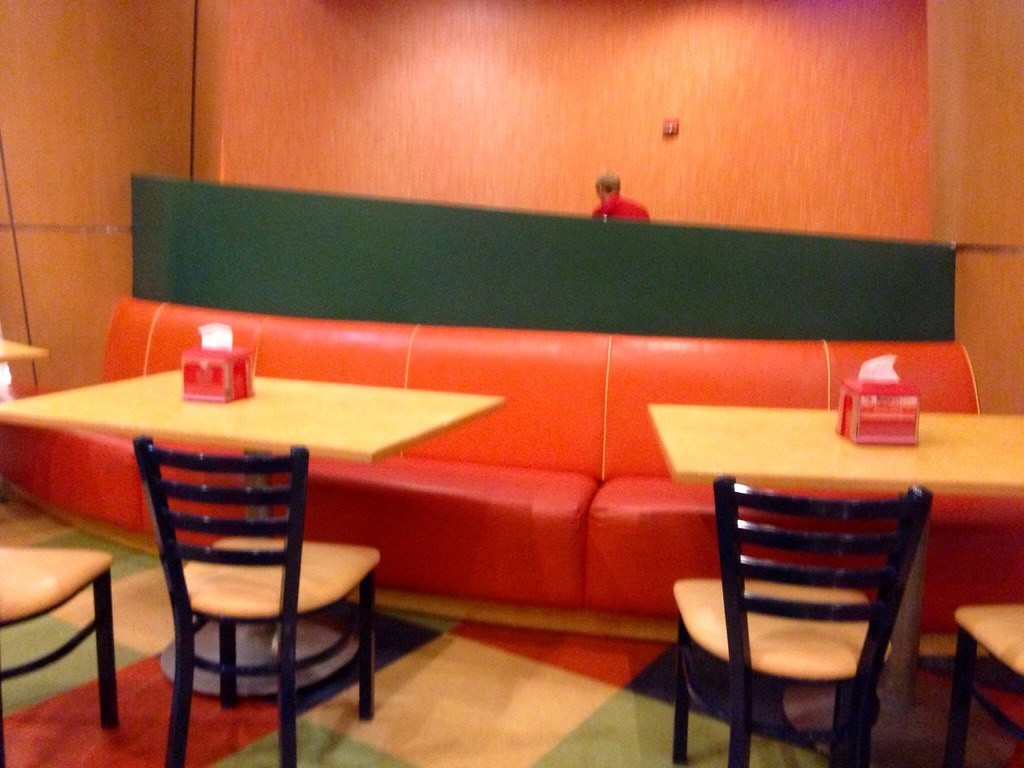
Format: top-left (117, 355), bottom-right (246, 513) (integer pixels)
top-left (134, 437), bottom-right (381, 768)
top-left (0, 547), bottom-right (119, 768)
top-left (673, 475), bottom-right (932, 768)
top-left (945, 605), bottom-right (1023, 768)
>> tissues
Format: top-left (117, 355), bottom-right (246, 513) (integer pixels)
top-left (182, 321), bottom-right (256, 403)
top-left (835, 353), bottom-right (920, 445)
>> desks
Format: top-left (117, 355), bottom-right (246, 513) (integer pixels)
top-left (648, 403), bottom-right (1023, 767)
top-left (0, 371), bottom-right (505, 698)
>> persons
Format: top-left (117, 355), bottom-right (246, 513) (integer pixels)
top-left (592, 169), bottom-right (651, 222)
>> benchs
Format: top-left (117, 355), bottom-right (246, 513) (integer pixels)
top-left (0, 299), bottom-right (1024, 632)
